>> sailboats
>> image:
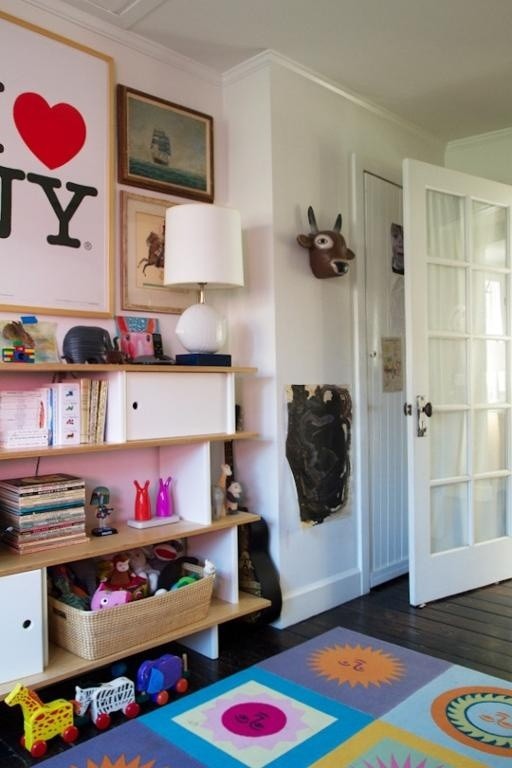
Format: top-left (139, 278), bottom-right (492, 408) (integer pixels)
top-left (150, 126), bottom-right (172, 166)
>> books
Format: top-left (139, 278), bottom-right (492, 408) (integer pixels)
top-left (113, 314), bottom-right (164, 358)
top-left (0, 377), bottom-right (111, 556)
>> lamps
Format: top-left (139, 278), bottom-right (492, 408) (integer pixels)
top-left (164, 203), bottom-right (245, 367)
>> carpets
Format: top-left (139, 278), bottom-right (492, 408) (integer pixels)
top-left (30, 624), bottom-right (511, 768)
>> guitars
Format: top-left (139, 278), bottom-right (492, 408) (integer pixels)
top-left (223, 404), bottom-right (282, 632)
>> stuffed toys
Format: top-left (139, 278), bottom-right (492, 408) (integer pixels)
top-left (221, 480), bottom-right (243, 516)
top-left (44, 537), bottom-right (205, 624)
top-left (216, 461), bottom-right (234, 518)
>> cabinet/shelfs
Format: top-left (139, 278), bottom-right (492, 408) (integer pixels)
top-left (1, 359), bottom-right (272, 699)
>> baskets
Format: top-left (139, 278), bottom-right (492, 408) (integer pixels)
top-left (48, 562), bottom-right (217, 661)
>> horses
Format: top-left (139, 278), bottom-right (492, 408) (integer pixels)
top-left (137, 232), bottom-right (164, 277)
